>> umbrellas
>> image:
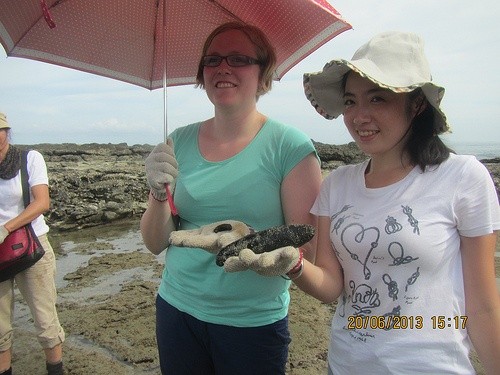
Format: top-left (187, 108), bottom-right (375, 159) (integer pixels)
top-left (1, 0), bottom-right (354, 216)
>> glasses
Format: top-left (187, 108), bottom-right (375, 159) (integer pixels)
top-left (201, 54), bottom-right (261, 67)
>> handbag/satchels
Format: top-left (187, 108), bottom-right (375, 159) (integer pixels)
top-left (0, 221), bottom-right (46, 283)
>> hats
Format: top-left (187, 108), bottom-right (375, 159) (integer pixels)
top-left (0, 111), bottom-right (12, 129)
top-left (303, 29), bottom-right (455, 135)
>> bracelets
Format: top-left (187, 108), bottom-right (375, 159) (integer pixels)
top-left (282, 260), bottom-right (304, 280)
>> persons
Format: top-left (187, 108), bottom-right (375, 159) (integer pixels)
top-left (224, 31), bottom-right (500, 375)
top-left (140, 21), bottom-right (323, 375)
top-left (0, 112), bottom-right (68, 375)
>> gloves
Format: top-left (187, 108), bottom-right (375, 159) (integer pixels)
top-left (223, 245), bottom-right (303, 279)
top-left (144, 136), bottom-right (179, 203)
top-left (168, 219), bottom-right (256, 254)
top-left (0, 225), bottom-right (9, 244)
